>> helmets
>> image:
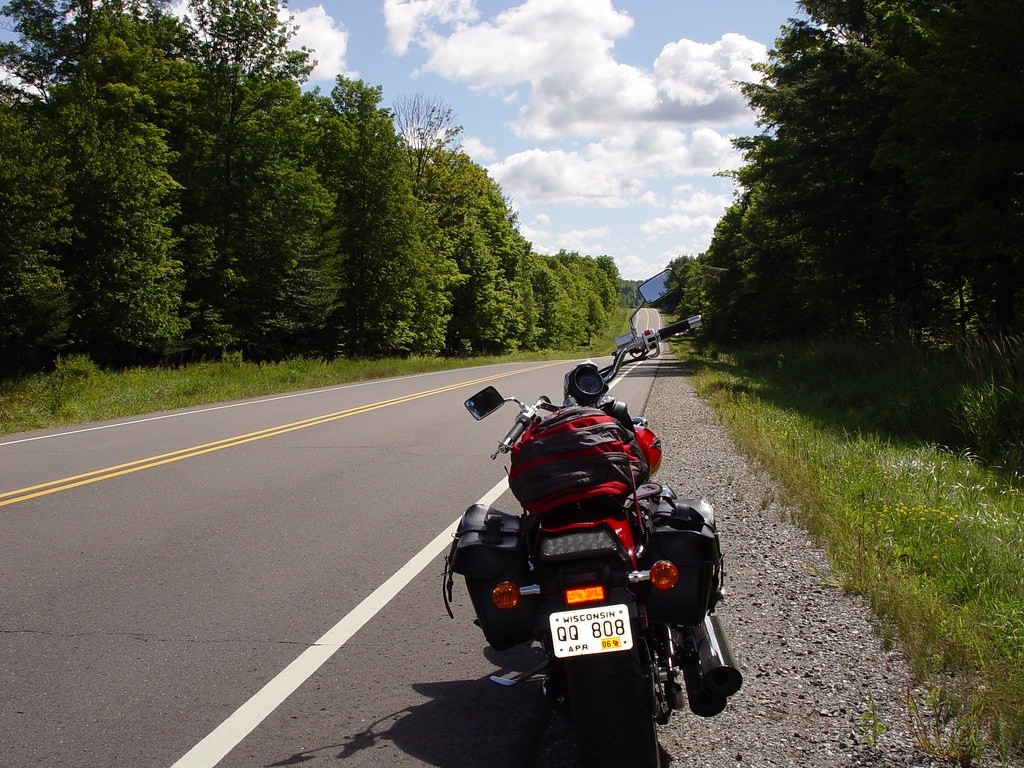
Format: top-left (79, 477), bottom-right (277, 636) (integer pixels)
top-left (632, 422), bottom-right (662, 476)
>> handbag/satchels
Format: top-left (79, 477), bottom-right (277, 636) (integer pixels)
top-left (509, 409), bottom-right (650, 523)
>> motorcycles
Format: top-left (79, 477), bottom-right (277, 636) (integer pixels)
top-left (442, 268), bottom-right (743, 768)
